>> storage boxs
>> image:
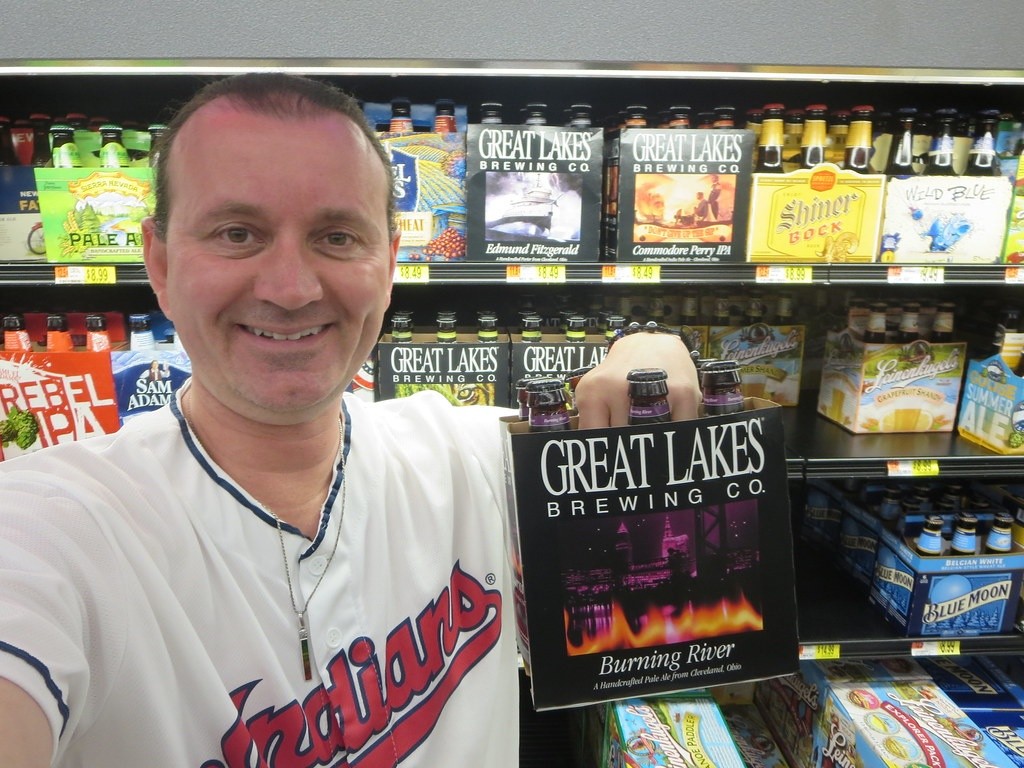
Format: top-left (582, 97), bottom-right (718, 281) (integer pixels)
top-left (0, 100), bottom-right (1024, 264)
top-left (0, 285), bottom-right (1024, 768)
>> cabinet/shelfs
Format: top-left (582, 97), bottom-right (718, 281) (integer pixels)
top-left (0, 61), bottom-right (1024, 673)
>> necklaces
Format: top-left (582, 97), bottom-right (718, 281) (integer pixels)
top-left (184, 384), bottom-right (346, 679)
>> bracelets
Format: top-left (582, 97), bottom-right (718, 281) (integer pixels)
top-left (606, 326), bottom-right (695, 355)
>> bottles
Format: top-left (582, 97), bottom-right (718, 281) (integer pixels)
top-left (0, 100), bottom-right (1024, 558)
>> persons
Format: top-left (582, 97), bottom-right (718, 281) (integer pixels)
top-left (0, 72), bottom-right (704, 768)
